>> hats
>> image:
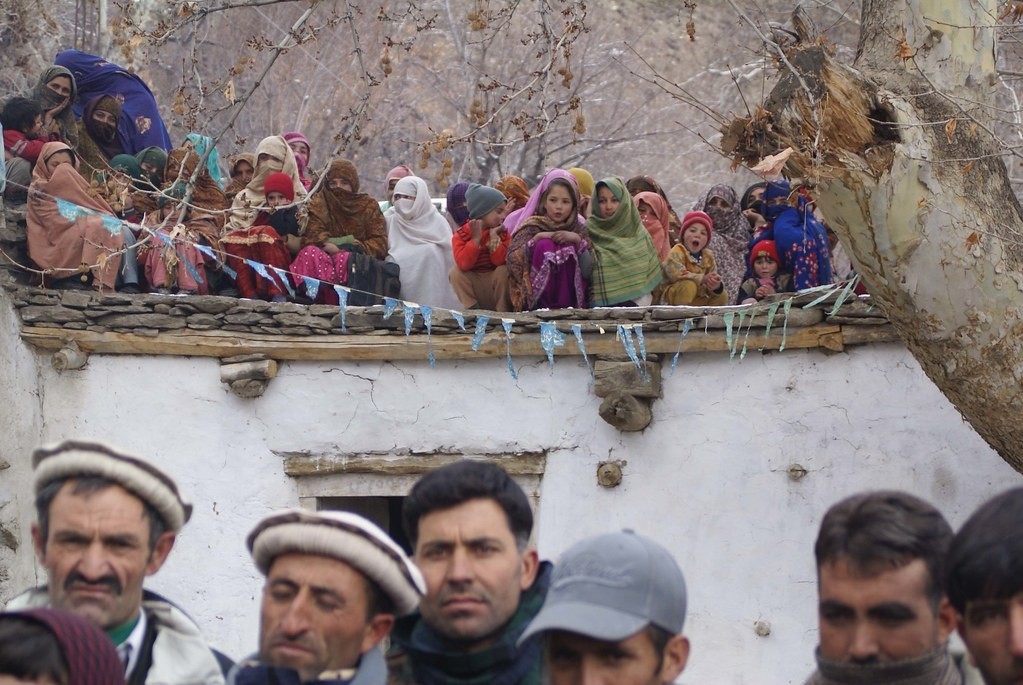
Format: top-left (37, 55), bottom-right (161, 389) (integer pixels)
top-left (29, 439), bottom-right (194, 541)
top-left (157, 182), bottom-right (191, 208)
top-left (515, 528), bottom-right (687, 648)
top-left (265, 173), bottom-right (294, 201)
top-left (465, 183), bottom-right (508, 219)
top-left (751, 239), bottom-right (782, 269)
top-left (678, 210), bottom-right (712, 249)
top-left (244, 507), bottom-right (427, 617)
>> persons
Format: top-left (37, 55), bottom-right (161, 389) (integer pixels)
top-left (941, 487), bottom-right (1023, 685)
top-left (388, 460), bottom-right (554, 685)
top-left (0, 49), bottom-right (834, 312)
top-left (517, 528), bottom-right (691, 685)
top-left (803, 489), bottom-right (986, 685)
top-left (226, 507), bottom-right (427, 685)
top-left (6, 438), bottom-right (226, 685)
top-left (0, 607), bottom-right (126, 685)
